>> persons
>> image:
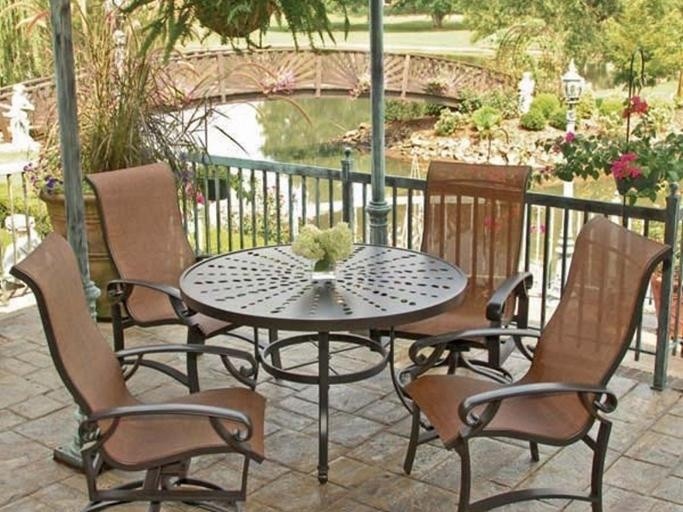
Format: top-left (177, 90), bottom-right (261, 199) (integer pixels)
top-left (3, 215), bottom-right (43, 301)
top-left (9, 83), bottom-right (34, 134)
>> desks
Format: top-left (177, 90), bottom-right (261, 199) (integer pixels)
top-left (177, 243), bottom-right (469, 485)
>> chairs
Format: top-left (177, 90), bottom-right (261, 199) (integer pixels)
top-left (400, 214), bottom-right (672, 512)
top-left (370, 159), bottom-right (535, 370)
top-left (9, 229), bottom-right (270, 512)
top-left (81, 161), bottom-right (285, 397)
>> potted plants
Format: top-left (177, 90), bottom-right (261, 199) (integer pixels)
top-left (1, 0), bottom-right (352, 322)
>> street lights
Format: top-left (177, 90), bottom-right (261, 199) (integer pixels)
top-left (551, 57), bottom-right (585, 291)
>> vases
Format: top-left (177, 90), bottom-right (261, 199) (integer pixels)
top-left (311, 255), bottom-right (337, 280)
top-left (652, 267), bottom-right (683, 341)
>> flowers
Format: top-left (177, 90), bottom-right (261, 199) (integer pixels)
top-left (290, 220), bottom-right (356, 272)
top-left (516, 68), bottom-right (683, 211)
